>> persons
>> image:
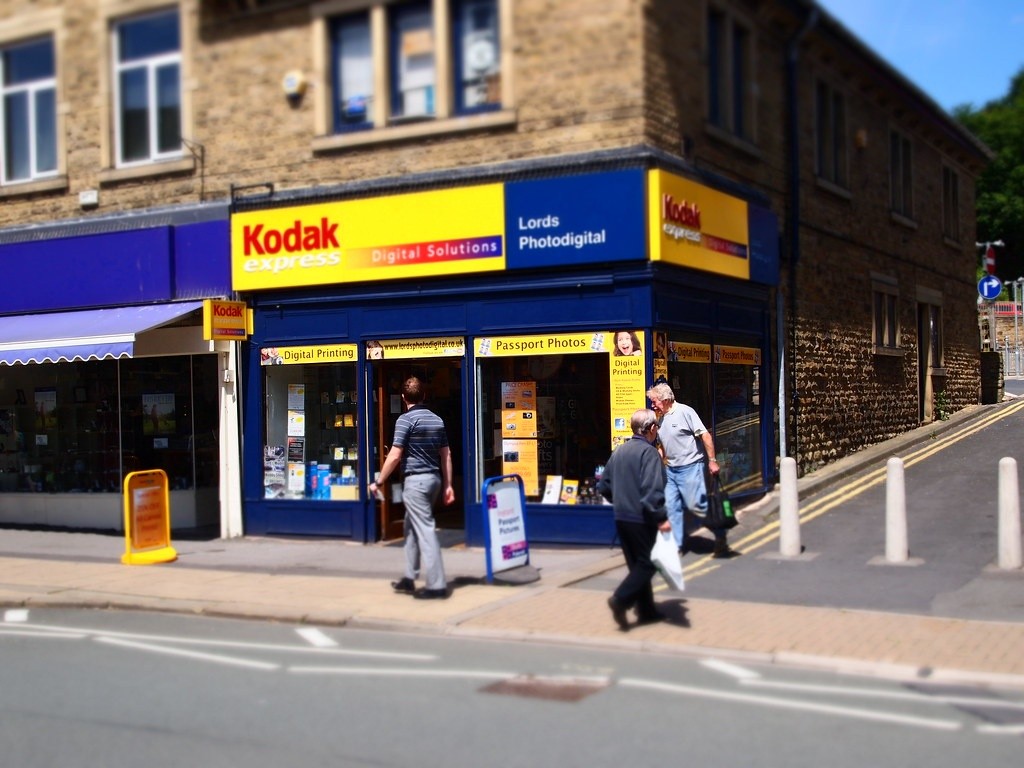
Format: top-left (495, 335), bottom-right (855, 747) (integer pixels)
top-left (151, 404), bottom-right (158, 434)
top-left (647, 384), bottom-right (729, 556)
top-left (591, 334), bottom-right (603, 350)
top-left (597, 409), bottom-right (671, 630)
top-left (367, 341), bottom-right (384, 359)
top-left (261, 348), bottom-right (279, 364)
top-left (370, 377), bottom-right (455, 598)
top-left (614, 331), bottom-right (642, 357)
top-left (653, 332), bottom-right (666, 359)
top-left (40, 402), bottom-right (47, 434)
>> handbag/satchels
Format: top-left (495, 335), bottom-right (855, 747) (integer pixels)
top-left (705, 472), bottom-right (739, 530)
top-left (650, 528), bottom-right (685, 592)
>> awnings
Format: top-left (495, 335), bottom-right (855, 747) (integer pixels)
top-left (0, 300), bottom-right (218, 367)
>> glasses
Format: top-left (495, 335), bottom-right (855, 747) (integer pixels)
top-left (655, 424), bottom-right (661, 429)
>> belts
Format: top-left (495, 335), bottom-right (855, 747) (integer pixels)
top-left (403, 469), bottom-right (439, 478)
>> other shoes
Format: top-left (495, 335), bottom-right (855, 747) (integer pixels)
top-left (607, 596), bottom-right (629, 629)
top-left (412, 586), bottom-right (447, 599)
top-left (390, 577), bottom-right (415, 592)
top-left (633, 606), bottom-right (668, 622)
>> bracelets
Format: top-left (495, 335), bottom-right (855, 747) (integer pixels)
top-left (709, 458), bottom-right (716, 462)
top-left (375, 481), bottom-right (383, 487)
